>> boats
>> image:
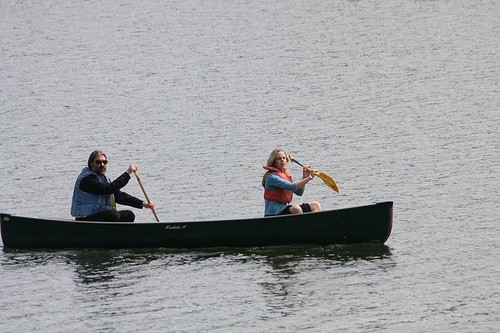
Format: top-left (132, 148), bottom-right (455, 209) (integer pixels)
top-left (0, 199), bottom-right (395, 251)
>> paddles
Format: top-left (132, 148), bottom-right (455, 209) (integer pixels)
top-left (289, 154), bottom-right (339, 193)
top-left (133, 169), bottom-right (160, 222)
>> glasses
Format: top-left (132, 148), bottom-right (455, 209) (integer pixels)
top-left (93, 160), bottom-right (108, 165)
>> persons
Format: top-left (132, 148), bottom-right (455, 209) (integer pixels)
top-left (70, 149), bottom-right (156, 223)
top-left (261, 148), bottom-right (321, 217)
